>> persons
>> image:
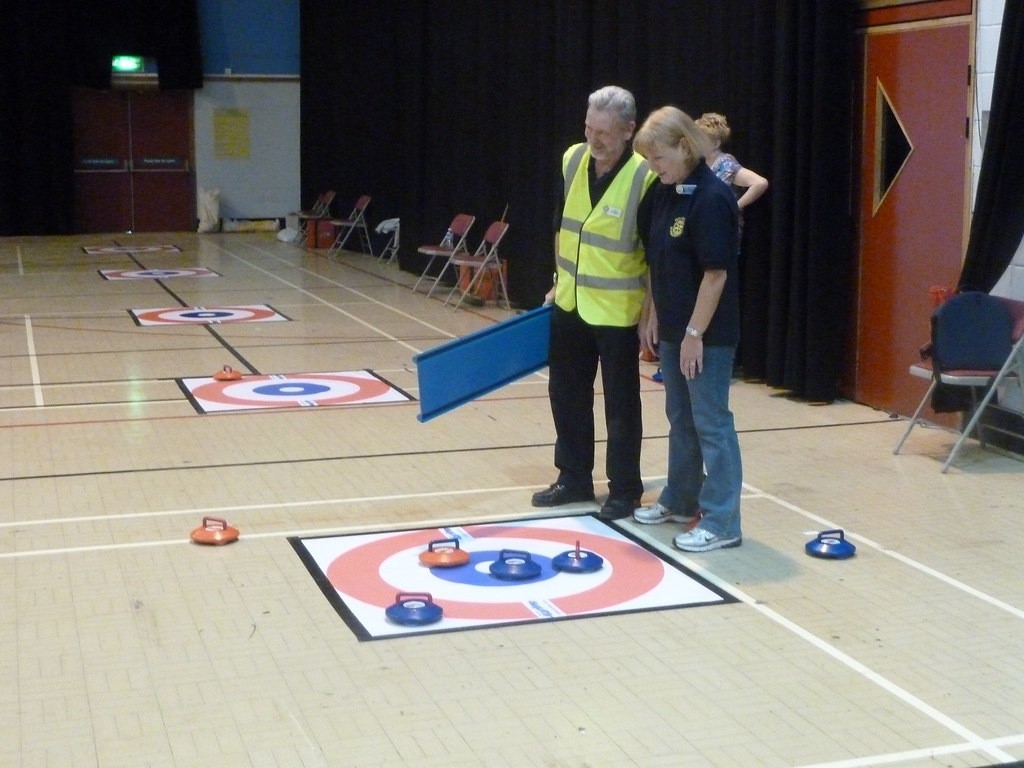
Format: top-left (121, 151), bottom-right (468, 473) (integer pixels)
top-left (532, 85), bottom-right (768, 552)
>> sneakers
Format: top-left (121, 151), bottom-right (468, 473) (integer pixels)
top-left (599, 494), bottom-right (641, 521)
top-left (531, 482), bottom-right (595, 508)
top-left (633, 501), bottom-right (700, 525)
top-left (672, 526), bottom-right (742, 552)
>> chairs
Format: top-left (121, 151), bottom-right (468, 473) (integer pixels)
top-left (294, 190), bottom-right (335, 246)
top-left (327, 196), bottom-right (373, 257)
top-left (443, 220), bottom-right (511, 312)
top-left (895, 238), bottom-right (1024, 475)
top-left (411, 215), bottom-right (475, 297)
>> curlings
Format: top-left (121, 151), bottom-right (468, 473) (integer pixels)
top-left (192, 513), bottom-right (242, 547)
top-left (551, 538), bottom-right (605, 575)
top-left (806, 530), bottom-right (859, 560)
top-left (213, 364), bottom-right (242, 382)
top-left (386, 591), bottom-right (444, 629)
top-left (652, 366), bottom-right (664, 383)
top-left (418, 537), bottom-right (472, 570)
top-left (487, 546), bottom-right (542, 581)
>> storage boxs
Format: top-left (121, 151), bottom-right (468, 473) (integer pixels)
top-left (307, 217), bottom-right (334, 249)
top-left (459, 257), bottom-right (507, 300)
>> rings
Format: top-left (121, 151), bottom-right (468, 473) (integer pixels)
top-left (690, 362), bottom-right (696, 366)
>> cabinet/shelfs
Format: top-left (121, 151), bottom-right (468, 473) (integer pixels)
top-left (69, 75), bottom-right (193, 234)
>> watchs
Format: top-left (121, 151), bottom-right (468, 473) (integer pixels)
top-left (552, 273), bottom-right (557, 284)
top-left (686, 327), bottom-right (700, 338)
